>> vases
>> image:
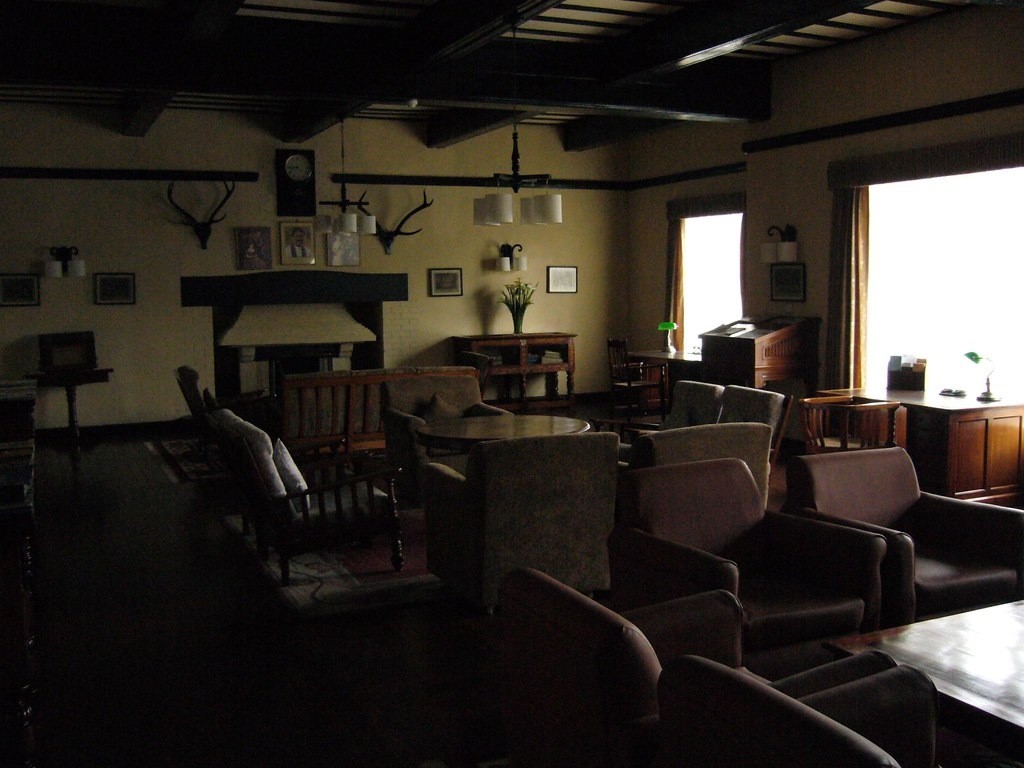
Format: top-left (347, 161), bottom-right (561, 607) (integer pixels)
top-left (511, 308), bottom-right (525, 334)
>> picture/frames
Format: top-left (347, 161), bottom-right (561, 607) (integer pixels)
top-left (0, 273), bottom-right (41, 306)
top-left (429, 268), bottom-right (463, 296)
top-left (235, 226), bottom-right (272, 270)
top-left (93, 272), bottom-right (136, 305)
top-left (547, 266), bottom-right (578, 293)
top-left (770, 263), bottom-right (807, 302)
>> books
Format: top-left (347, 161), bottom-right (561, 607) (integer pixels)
top-left (0, 379), bottom-right (38, 518)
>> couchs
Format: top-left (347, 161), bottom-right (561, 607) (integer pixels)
top-left (381, 374), bottom-right (1024, 768)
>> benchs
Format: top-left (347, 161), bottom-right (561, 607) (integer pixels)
top-left (591, 379), bottom-right (794, 499)
top-left (205, 367), bottom-right (479, 585)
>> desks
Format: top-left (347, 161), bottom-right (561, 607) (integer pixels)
top-left (822, 600), bottom-right (1024, 766)
top-left (618, 350), bottom-right (702, 415)
top-left (416, 416), bottom-right (591, 458)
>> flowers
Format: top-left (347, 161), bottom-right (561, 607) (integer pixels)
top-left (497, 278), bottom-right (539, 313)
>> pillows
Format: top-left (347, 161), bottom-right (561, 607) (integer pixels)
top-left (422, 394), bottom-right (464, 424)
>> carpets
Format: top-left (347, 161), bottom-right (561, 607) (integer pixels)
top-left (155, 438), bottom-right (240, 481)
top-left (220, 495), bottom-right (444, 623)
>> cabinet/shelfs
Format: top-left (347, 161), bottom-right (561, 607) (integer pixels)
top-left (451, 332), bottom-right (578, 403)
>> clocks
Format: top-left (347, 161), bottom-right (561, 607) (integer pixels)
top-left (326, 233), bottom-right (362, 267)
top-left (279, 221), bottom-right (316, 265)
top-left (275, 149), bottom-right (316, 216)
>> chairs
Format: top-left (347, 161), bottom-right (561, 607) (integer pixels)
top-left (798, 395), bottom-right (900, 456)
top-left (451, 350), bottom-right (496, 400)
top-left (607, 337), bottom-right (669, 425)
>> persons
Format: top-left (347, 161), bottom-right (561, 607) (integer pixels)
top-left (285, 227), bottom-right (311, 257)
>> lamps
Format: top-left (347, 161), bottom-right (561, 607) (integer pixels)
top-left (657, 321), bottom-right (678, 353)
top-left (496, 244), bottom-right (527, 272)
top-left (759, 224), bottom-right (799, 264)
top-left (314, 119), bottom-right (376, 235)
top-left (965, 352), bottom-right (999, 401)
top-left (44, 246), bottom-right (86, 278)
top-left (473, 123), bottom-right (562, 227)
